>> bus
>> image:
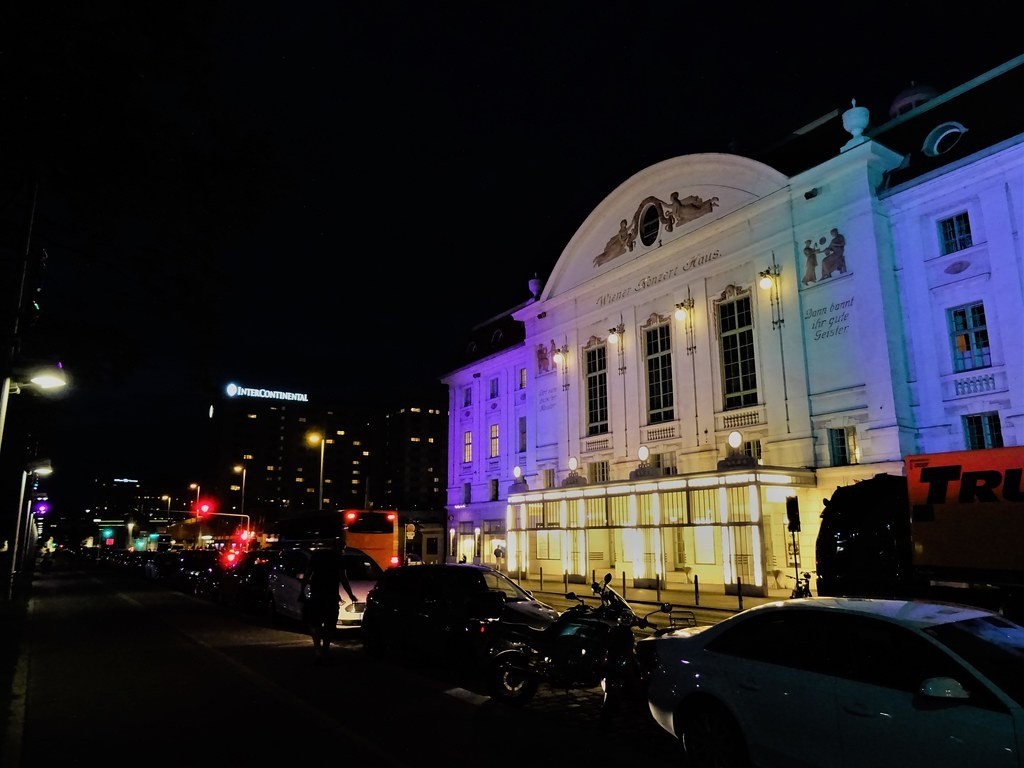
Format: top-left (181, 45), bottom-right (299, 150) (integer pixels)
top-left (268, 507), bottom-right (400, 573)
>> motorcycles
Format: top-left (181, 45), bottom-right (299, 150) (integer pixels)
top-left (442, 575), bottom-right (671, 707)
top-left (564, 582), bottom-right (677, 694)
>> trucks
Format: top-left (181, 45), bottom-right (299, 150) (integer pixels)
top-left (816, 446), bottom-right (1024, 627)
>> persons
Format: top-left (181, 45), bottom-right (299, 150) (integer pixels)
top-left (537, 340), bottom-right (559, 374)
top-left (494, 545), bottom-right (503, 572)
top-left (459, 553), bottom-right (466, 564)
top-left (659, 192), bottom-right (719, 227)
top-left (299, 539), bottom-right (357, 656)
top-left (593, 216), bottom-right (636, 268)
top-left (800, 229), bottom-right (845, 286)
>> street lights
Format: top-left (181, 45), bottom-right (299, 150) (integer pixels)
top-left (127, 522), bottom-right (136, 551)
top-left (7, 465), bottom-right (54, 600)
top-left (161, 495), bottom-right (172, 534)
top-left (305, 431), bottom-right (325, 509)
top-left (0, 366), bottom-right (72, 450)
top-left (233, 465), bottom-right (246, 532)
top-left (190, 483), bottom-right (200, 551)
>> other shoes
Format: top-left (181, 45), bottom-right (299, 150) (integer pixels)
top-left (314, 656), bottom-right (336, 667)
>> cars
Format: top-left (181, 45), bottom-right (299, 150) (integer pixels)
top-left (39, 547), bottom-right (383, 639)
top-left (360, 564), bottom-right (561, 696)
top-left (637, 596), bottom-right (1024, 768)
